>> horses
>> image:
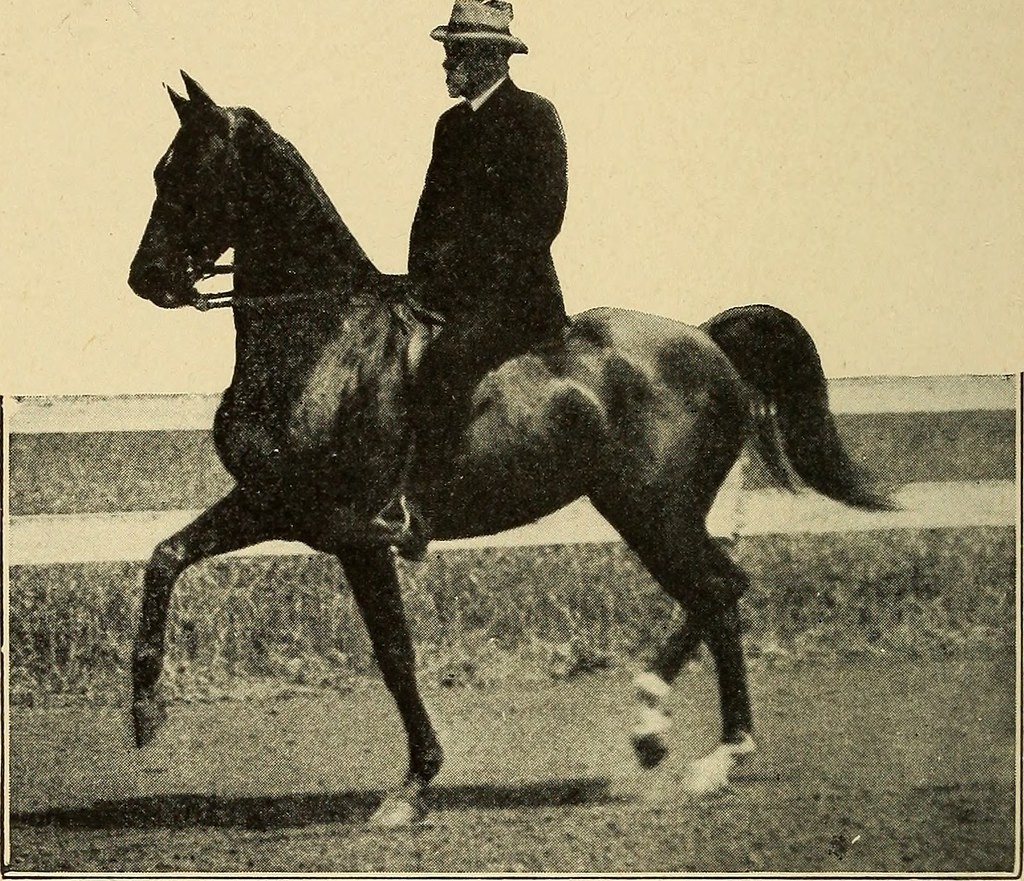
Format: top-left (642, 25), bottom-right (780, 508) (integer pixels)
top-left (127, 68), bottom-right (905, 829)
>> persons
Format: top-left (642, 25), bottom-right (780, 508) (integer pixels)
top-left (370, 0), bottom-right (569, 562)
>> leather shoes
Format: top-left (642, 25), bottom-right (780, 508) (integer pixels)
top-left (364, 508), bottom-right (433, 563)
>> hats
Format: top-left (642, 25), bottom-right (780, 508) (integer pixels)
top-left (430, 0), bottom-right (529, 55)
